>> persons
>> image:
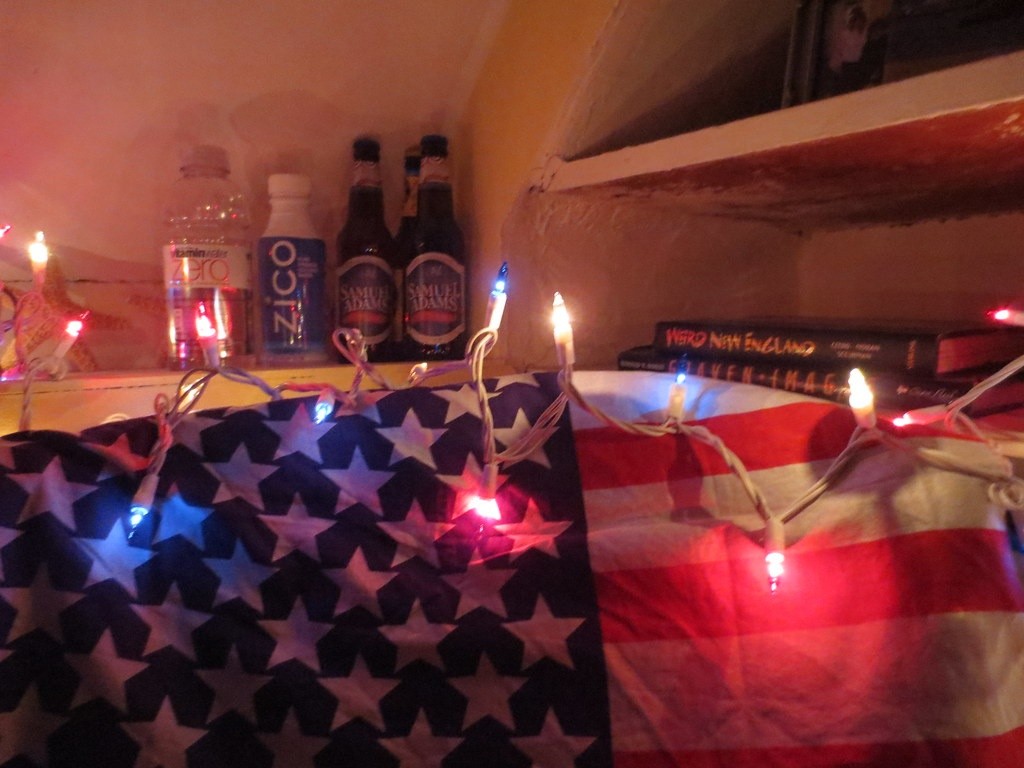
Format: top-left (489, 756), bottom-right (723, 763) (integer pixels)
top-left (821, 0), bottom-right (888, 99)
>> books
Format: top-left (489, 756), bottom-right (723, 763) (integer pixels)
top-left (617, 315), bottom-right (1024, 419)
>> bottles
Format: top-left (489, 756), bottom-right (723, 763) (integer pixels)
top-left (334, 134), bottom-right (400, 363)
top-left (392, 146), bottom-right (422, 362)
top-left (160, 145), bottom-right (251, 371)
top-left (402, 134), bottom-right (469, 362)
top-left (251, 173), bottom-right (334, 367)
